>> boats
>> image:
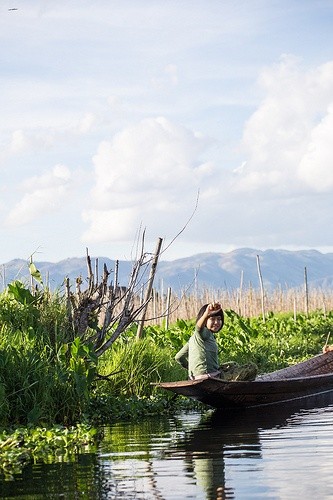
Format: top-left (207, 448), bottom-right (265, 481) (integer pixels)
top-left (148, 344), bottom-right (333, 414)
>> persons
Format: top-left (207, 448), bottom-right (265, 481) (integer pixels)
top-left (174, 299), bottom-right (258, 382)
